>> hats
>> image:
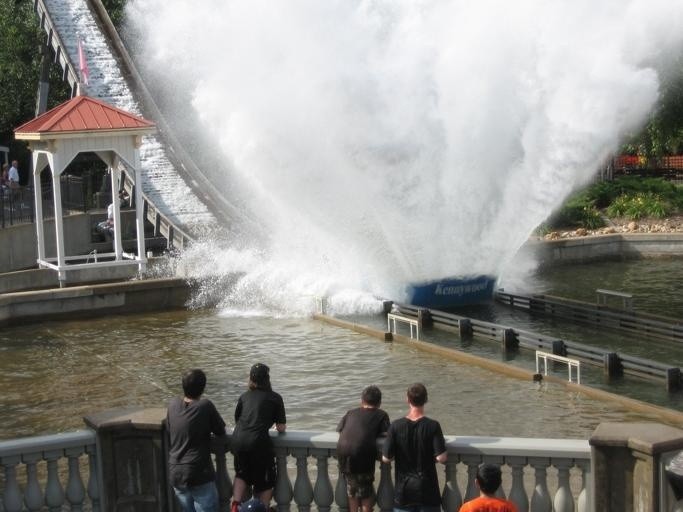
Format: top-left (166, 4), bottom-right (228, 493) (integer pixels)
top-left (251, 363), bottom-right (273, 376)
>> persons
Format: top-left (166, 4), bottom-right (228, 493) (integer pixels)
top-left (0, 159), bottom-right (23, 210)
top-left (381, 383), bottom-right (447, 512)
top-left (456, 462), bottom-right (520, 512)
top-left (335, 384), bottom-right (390, 512)
top-left (161, 369), bottom-right (226, 512)
top-left (228, 362), bottom-right (287, 511)
top-left (106, 188), bottom-right (130, 226)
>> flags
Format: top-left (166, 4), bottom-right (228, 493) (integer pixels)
top-left (77, 41), bottom-right (89, 87)
